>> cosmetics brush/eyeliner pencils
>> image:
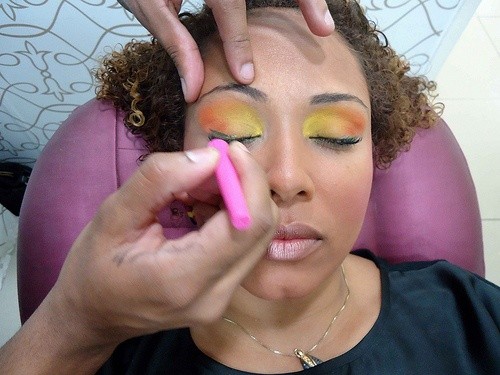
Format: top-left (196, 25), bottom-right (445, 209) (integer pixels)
top-left (207, 138), bottom-right (252, 230)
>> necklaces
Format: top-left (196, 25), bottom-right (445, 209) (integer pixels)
top-left (224, 264), bottom-right (351, 369)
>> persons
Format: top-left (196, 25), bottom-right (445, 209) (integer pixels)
top-left (93, 0), bottom-right (500, 375)
top-left (0, 0), bottom-right (336, 375)
